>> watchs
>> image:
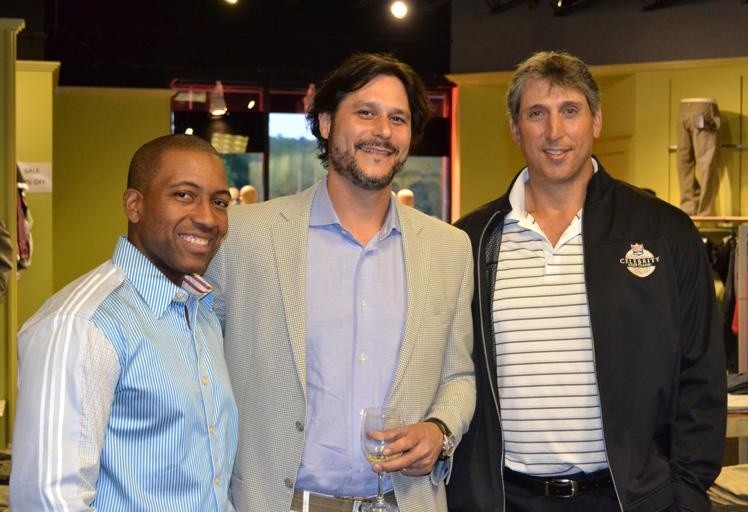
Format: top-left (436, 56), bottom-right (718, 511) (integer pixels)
top-left (424, 418), bottom-right (456, 462)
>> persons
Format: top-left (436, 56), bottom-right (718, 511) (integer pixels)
top-left (195, 53), bottom-right (482, 511)
top-left (446, 48), bottom-right (728, 511)
top-left (9, 133), bottom-right (240, 510)
top-left (675, 97), bottom-right (721, 218)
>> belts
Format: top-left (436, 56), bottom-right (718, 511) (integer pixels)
top-left (290, 489), bottom-right (398, 512)
top-left (503, 467), bottom-right (613, 499)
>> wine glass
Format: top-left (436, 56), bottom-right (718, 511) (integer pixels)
top-left (358, 408), bottom-right (406, 512)
